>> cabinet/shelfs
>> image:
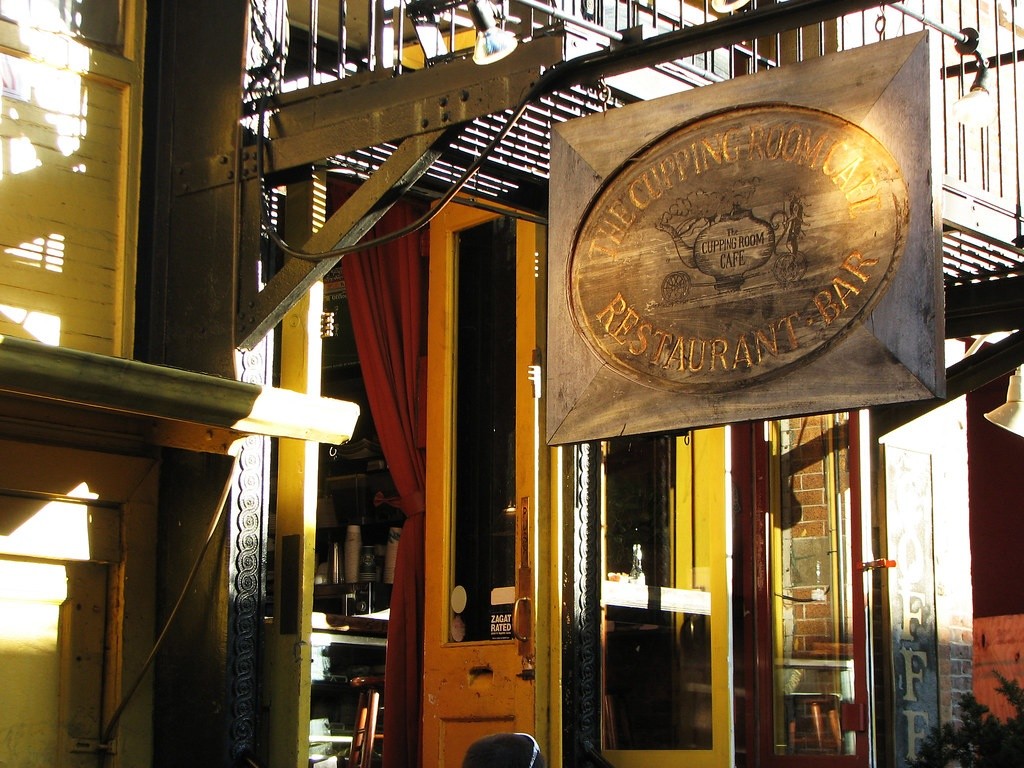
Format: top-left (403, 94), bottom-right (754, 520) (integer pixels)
top-left (308, 632), bottom-right (388, 768)
top-left (310, 454), bottom-right (405, 600)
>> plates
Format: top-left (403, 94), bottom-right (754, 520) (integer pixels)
top-left (269, 514), bottom-right (276, 531)
top-left (336, 438), bottom-right (381, 460)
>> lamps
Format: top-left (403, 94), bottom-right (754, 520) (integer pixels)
top-left (467, 0), bottom-right (518, 66)
top-left (955, 28), bottom-right (994, 129)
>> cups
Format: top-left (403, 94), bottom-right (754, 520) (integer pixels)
top-left (358, 546), bottom-right (376, 582)
top-left (382, 527), bottom-right (402, 584)
top-left (344, 525), bottom-right (362, 583)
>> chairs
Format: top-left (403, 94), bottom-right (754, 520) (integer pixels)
top-left (350, 688), bottom-right (380, 768)
